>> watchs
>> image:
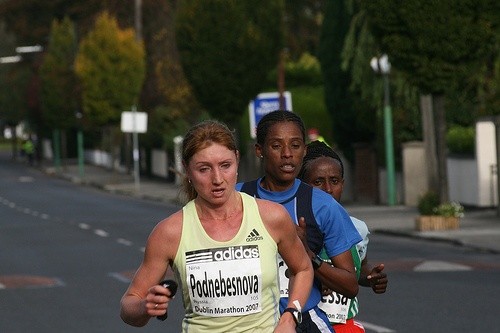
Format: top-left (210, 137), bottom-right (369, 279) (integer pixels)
top-left (283, 308), bottom-right (303, 328)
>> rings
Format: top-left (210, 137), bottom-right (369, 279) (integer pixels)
top-left (154, 304), bottom-right (158, 309)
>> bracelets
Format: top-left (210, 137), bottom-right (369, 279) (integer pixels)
top-left (311, 253), bottom-right (323, 270)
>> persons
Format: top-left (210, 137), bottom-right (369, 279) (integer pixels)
top-left (235, 111), bottom-right (363, 333)
top-left (119, 119), bottom-right (315, 333)
top-left (21, 137), bottom-right (35, 166)
top-left (295, 141), bottom-right (388, 333)
top-left (305, 128), bottom-right (330, 148)
